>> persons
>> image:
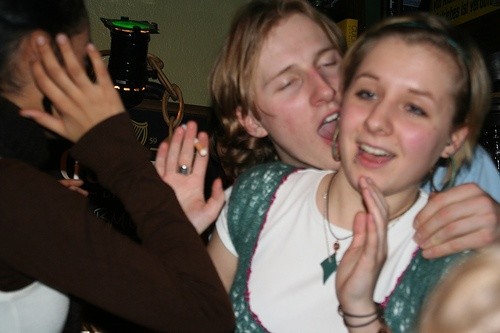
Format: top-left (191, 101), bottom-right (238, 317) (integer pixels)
top-left (155, 14), bottom-right (500, 333)
top-left (0, 0), bottom-right (239, 333)
top-left (207, 0), bottom-right (500, 259)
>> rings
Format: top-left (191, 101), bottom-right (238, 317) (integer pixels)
top-left (177, 165), bottom-right (188, 175)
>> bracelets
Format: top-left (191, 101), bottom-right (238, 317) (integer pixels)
top-left (337, 301), bottom-right (391, 333)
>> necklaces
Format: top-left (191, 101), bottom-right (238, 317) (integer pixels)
top-left (319, 169), bottom-right (421, 284)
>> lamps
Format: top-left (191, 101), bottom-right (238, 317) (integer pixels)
top-left (101, 15), bottom-right (167, 109)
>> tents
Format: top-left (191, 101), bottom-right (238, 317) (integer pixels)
top-left (336, 18), bottom-right (358, 53)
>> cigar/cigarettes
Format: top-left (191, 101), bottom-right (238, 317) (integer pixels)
top-left (182, 124), bottom-right (208, 157)
